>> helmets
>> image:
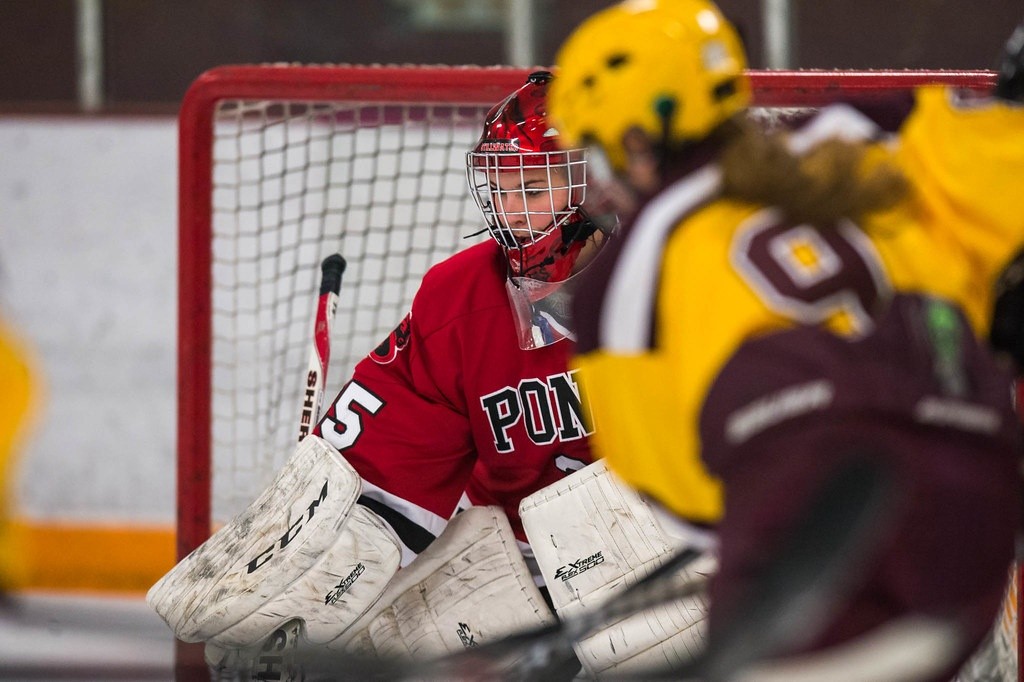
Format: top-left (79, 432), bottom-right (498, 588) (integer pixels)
top-left (465, 72), bottom-right (599, 300)
top-left (549, 0), bottom-right (754, 167)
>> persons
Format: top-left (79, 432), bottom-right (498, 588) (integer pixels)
top-left (146, 0), bottom-right (1024, 682)
